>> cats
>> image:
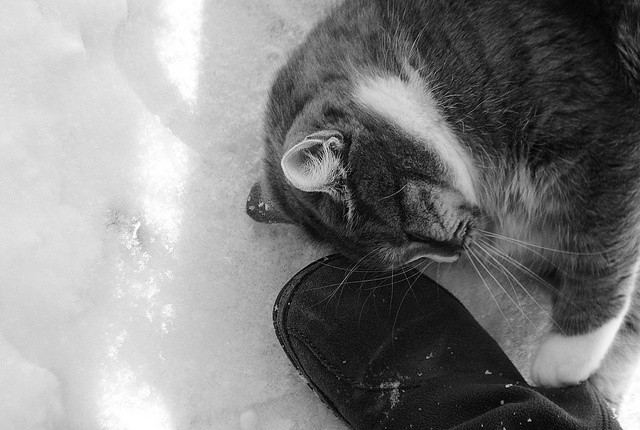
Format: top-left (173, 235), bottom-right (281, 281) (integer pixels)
top-left (245, 1), bottom-right (639, 388)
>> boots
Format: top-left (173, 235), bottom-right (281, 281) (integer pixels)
top-left (271, 252), bottom-right (623, 430)
